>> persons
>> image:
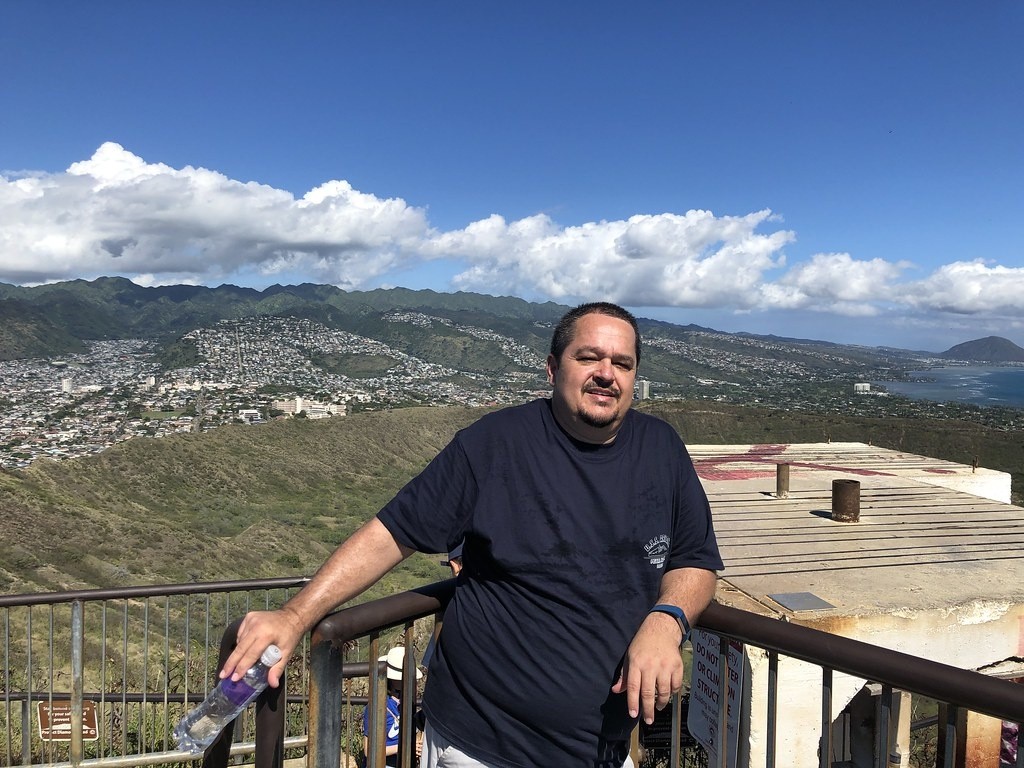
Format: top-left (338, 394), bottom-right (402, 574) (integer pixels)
top-left (362, 646), bottom-right (423, 768)
top-left (217, 301), bottom-right (725, 768)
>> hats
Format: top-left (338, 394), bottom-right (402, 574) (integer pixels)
top-left (377, 646), bottom-right (423, 681)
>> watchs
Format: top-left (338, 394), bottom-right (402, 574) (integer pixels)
top-left (648, 604), bottom-right (692, 647)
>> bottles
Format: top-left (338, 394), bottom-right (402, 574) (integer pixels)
top-left (172, 645), bottom-right (281, 755)
